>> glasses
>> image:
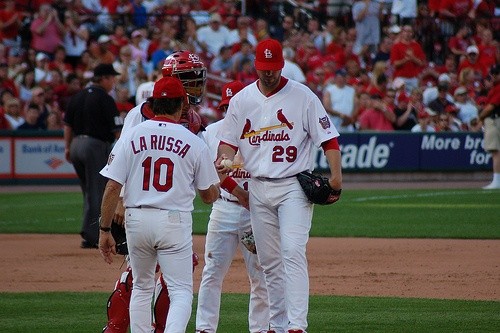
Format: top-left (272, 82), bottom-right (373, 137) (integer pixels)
top-left (468, 52), bottom-right (476, 56)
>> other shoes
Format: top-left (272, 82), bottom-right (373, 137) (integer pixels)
top-left (481, 179), bottom-right (500, 189)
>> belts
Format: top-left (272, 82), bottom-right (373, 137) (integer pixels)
top-left (258, 175), bottom-right (297, 180)
top-left (218, 194), bottom-right (239, 203)
top-left (77, 135), bottom-right (89, 138)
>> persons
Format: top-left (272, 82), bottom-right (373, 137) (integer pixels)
top-left (214, 39), bottom-right (342, 333)
top-left (0, 0), bottom-right (500, 333)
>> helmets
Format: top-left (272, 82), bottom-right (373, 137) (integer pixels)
top-left (162, 50), bottom-right (207, 105)
top-left (135, 81), bottom-right (156, 106)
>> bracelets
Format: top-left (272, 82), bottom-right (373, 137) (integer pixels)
top-left (100, 227), bottom-right (111, 232)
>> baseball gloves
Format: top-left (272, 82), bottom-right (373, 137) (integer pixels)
top-left (98, 217), bottom-right (129, 255)
top-left (241, 229), bottom-right (257, 254)
top-left (296, 171), bottom-right (341, 205)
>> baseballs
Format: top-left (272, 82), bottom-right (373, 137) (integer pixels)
top-left (220, 159), bottom-right (232, 171)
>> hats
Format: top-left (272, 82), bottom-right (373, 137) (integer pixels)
top-left (220, 46), bottom-right (232, 54)
top-left (255, 39), bottom-right (285, 71)
top-left (218, 79), bottom-right (244, 109)
top-left (396, 46), bottom-right (478, 113)
top-left (98, 35), bottom-right (112, 43)
top-left (36, 52), bottom-right (48, 61)
top-left (32, 87), bottom-right (45, 96)
top-left (211, 13), bottom-right (221, 23)
top-left (132, 31), bottom-right (142, 39)
top-left (120, 47), bottom-right (132, 57)
top-left (94, 63), bottom-right (122, 76)
top-left (152, 76), bottom-right (189, 105)
